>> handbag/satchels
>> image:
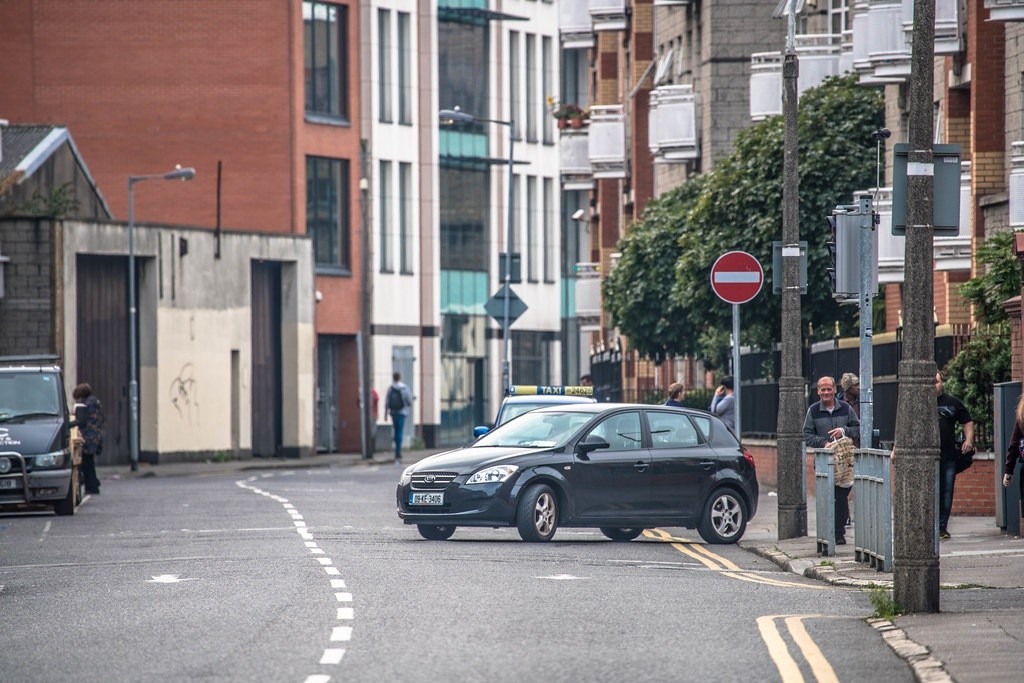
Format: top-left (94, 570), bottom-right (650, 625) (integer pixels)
top-left (956, 440), bottom-right (974, 474)
top-left (826, 430), bottom-right (855, 488)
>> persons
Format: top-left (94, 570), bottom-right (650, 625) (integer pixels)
top-left (370, 389), bottom-right (380, 449)
top-left (1001, 395), bottom-right (1024, 519)
top-left (890, 370), bottom-right (974, 540)
top-left (665, 382), bottom-right (685, 408)
top-left (802, 376), bottom-right (861, 544)
top-left (838, 372), bottom-right (862, 419)
top-left (580, 375), bottom-right (607, 402)
top-left (710, 376), bottom-right (736, 436)
top-left (384, 372), bottom-right (414, 459)
top-left (71, 383), bottom-right (105, 495)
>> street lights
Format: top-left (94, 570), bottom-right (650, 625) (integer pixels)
top-left (439, 106), bottom-right (515, 399)
top-left (127, 165), bottom-right (196, 470)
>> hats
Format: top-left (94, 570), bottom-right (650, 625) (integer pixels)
top-left (841, 372), bottom-right (860, 393)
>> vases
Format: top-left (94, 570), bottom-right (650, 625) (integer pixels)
top-left (570, 116), bottom-right (583, 128)
top-left (558, 118), bottom-right (570, 129)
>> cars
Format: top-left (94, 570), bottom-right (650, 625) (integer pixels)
top-left (473, 386), bottom-right (605, 438)
top-left (396, 403), bottom-right (759, 544)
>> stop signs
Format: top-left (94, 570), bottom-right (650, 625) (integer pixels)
top-left (710, 251), bottom-right (764, 304)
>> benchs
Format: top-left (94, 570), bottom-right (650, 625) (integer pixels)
top-left (653, 425), bottom-right (697, 447)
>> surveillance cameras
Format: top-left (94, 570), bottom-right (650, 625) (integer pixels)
top-left (571, 209), bottom-right (586, 222)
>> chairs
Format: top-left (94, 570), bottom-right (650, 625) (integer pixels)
top-left (614, 418), bottom-right (636, 448)
top-left (568, 422), bottom-right (590, 437)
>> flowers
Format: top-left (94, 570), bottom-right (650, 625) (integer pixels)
top-left (547, 96), bottom-right (587, 119)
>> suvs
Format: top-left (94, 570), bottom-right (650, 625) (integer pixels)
top-left (0, 354), bottom-right (87, 515)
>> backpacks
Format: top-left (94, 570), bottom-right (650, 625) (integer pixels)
top-left (388, 385), bottom-right (406, 411)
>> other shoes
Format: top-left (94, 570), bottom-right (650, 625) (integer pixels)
top-left (835, 537), bottom-right (846, 546)
top-left (845, 522), bottom-right (852, 529)
top-left (939, 529), bottom-right (951, 539)
top-left (84, 490), bottom-right (100, 495)
